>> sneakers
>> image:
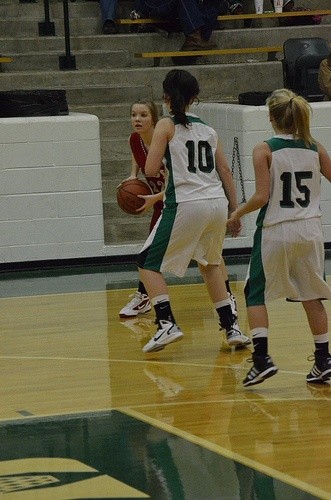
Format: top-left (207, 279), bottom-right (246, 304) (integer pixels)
top-left (226, 292), bottom-right (238, 316)
top-left (119, 292), bottom-right (152, 317)
top-left (283, 0), bottom-right (294, 12)
top-left (242, 355), bottom-right (278, 385)
top-left (220, 324), bottom-right (250, 345)
top-left (142, 319), bottom-right (184, 353)
top-left (229, 3), bottom-right (243, 15)
top-left (305, 352), bottom-right (331, 380)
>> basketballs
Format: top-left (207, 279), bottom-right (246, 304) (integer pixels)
top-left (117, 178), bottom-right (153, 214)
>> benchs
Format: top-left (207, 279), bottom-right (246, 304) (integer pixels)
top-left (135, 43), bottom-right (283, 66)
top-left (0, 57), bottom-right (13, 72)
top-left (115, 9), bottom-right (331, 33)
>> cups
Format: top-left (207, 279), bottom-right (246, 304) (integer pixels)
top-left (273, 0), bottom-right (283, 13)
top-left (254, 0), bottom-right (263, 15)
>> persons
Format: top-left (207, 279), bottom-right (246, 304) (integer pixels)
top-left (225, 88), bottom-right (331, 387)
top-left (117, 99), bottom-right (239, 318)
top-left (142, 69), bottom-right (251, 355)
top-left (100, 1), bottom-right (299, 51)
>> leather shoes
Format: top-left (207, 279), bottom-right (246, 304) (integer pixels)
top-left (102, 21), bottom-right (117, 34)
top-left (183, 36), bottom-right (217, 51)
